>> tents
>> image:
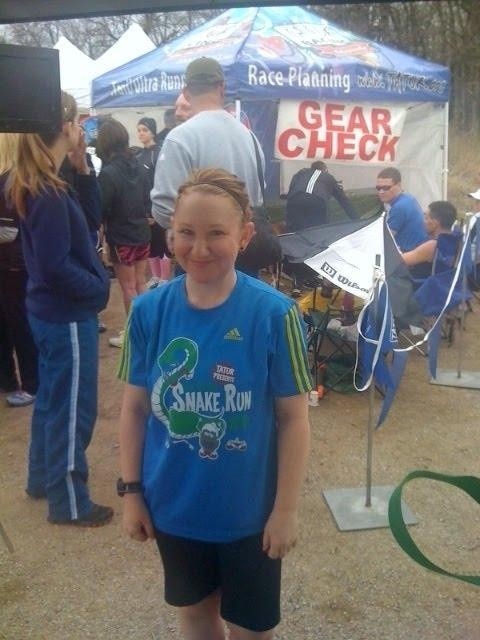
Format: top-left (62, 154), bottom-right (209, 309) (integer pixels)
top-left (90, 8), bottom-right (451, 229)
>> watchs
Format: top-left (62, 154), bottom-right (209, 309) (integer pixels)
top-left (116, 480), bottom-right (141, 494)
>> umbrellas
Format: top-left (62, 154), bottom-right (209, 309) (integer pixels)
top-left (269, 208), bottom-right (413, 374)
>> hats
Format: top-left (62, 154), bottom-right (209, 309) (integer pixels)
top-left (468, 188), bottom-right (480, 199)
top-left (186, 56), bottom-right (224, 83)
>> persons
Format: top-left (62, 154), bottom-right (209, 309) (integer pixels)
top-left (375, 170), bottom-right (425, 252)
top-left (394, 203), bottom-right (461, 291)
top-left (15, 88), bottom-right (114, 526)
top-left (151, 57), bottom-right (267, 278)
top-left (466, 186), bottom-right (480, 276)
top-left (286, 160), bottom-right (358, 231)
top-left (2, 127), bottom-right (35, 406)
top-left (88, 117), bottom-right (153, 349)
top-left (173, 92), bottom-right (194, 122)
top-left (137, 117), bottom-right (168, 288)
top-left (116, 170), bottom-right (314, 639)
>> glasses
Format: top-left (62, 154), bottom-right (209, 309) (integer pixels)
top-left (375, 183), bottom-right (397, 191)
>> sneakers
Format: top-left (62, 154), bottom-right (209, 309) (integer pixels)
top-left (144, 277), bottom-right (158, 288)
top-left (48, 499), bottom-right (115, 526)
top-left (109, 330), bottom-right (125, 347)
top-left (0, 389), bottom-right (35, 407)
